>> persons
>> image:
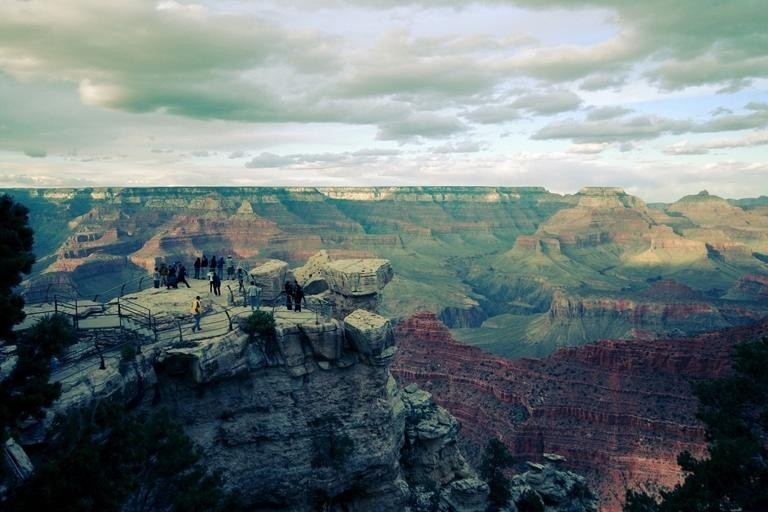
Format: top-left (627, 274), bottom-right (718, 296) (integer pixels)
top-left (151, 253), bottom-right (307, 313)
top-left (191, 295), bottom-right (203, 333)
